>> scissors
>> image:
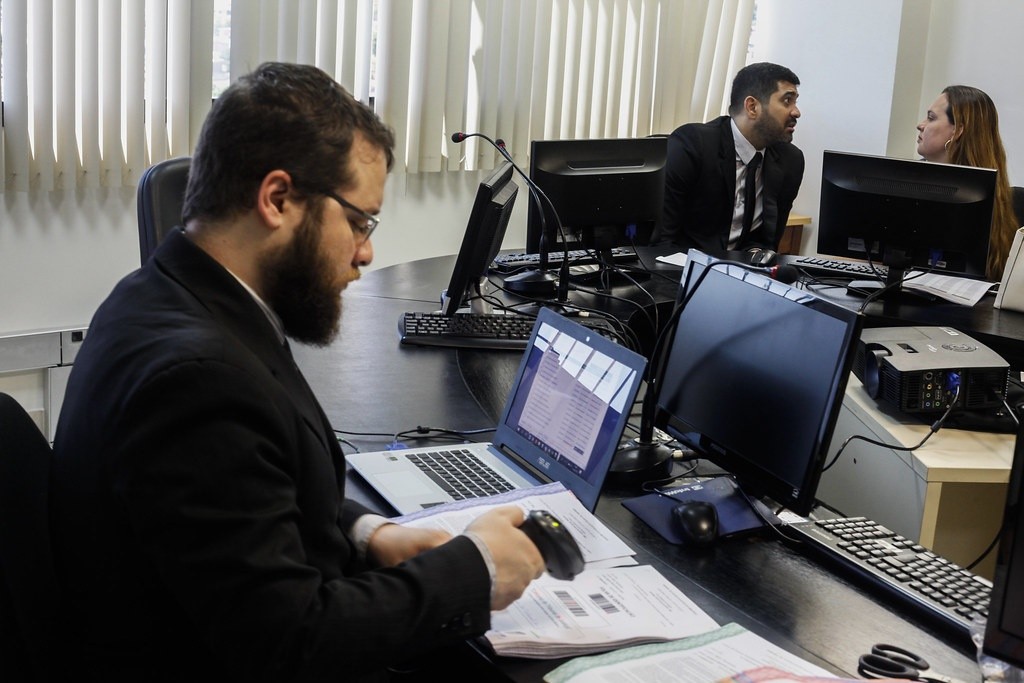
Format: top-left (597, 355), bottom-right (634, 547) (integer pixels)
top-left (859, 643), bottom-right (968, 683)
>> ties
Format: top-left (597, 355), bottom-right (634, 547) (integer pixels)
top-left (734, 152), bottom-right (762, 251)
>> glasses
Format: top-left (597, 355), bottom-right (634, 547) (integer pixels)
top-left (255, 173), bottom-right (380, 244)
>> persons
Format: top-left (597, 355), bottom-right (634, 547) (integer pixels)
top-left (19, 64), bottom-right (545, 683)
top-left (917, 83), bottom-right (1018, 279)
top-left (647, 62), bottom-right (806, 251)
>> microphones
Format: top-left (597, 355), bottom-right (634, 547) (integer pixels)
top-left (602, 261), bottom-right (800, 499)
top-left (452, 131), bottom-right (578, 319)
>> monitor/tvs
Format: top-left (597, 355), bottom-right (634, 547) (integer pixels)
top-left (526, 137), bottom-right (669, 285)
top-left (817, 150), bottom-right (998, 302)
top-left (983, 404), bottom-right (1024, 671)
top-left (439, 160), bottom-right (520, 316)
top-left (620, 248), bottom-right (866, 546)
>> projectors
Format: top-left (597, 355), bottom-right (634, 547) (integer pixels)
top-left (851, 326), bottom-right (1011, 422)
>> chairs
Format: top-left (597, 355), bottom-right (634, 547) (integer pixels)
top-left (0, 383), bottom-right (76, 683)
top-left (138, 158), bottom-right (191, 262)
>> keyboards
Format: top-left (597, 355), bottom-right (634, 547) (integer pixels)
top-left (787, 255), bottom-right (907, 283)
top-left (397, 310), bottom-right (618, 350)
top-left (494, 246), bottom-right (638, 273)
top-left (781, 515), bottom-right (994, 658)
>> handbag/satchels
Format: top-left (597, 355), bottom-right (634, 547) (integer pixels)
top-left (993, 226), bottom-right (1024, 313)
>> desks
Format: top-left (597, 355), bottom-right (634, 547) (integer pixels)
top-left (285, 212), bottom-right (1024, 683)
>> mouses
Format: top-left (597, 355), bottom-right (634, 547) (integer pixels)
top-left (671, 501), bottom-right (720, 541)
top-left (745, 248), bottom-right (777, 267)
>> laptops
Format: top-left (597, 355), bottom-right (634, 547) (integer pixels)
top-left (344, 307), bottom-right (649, 520)
